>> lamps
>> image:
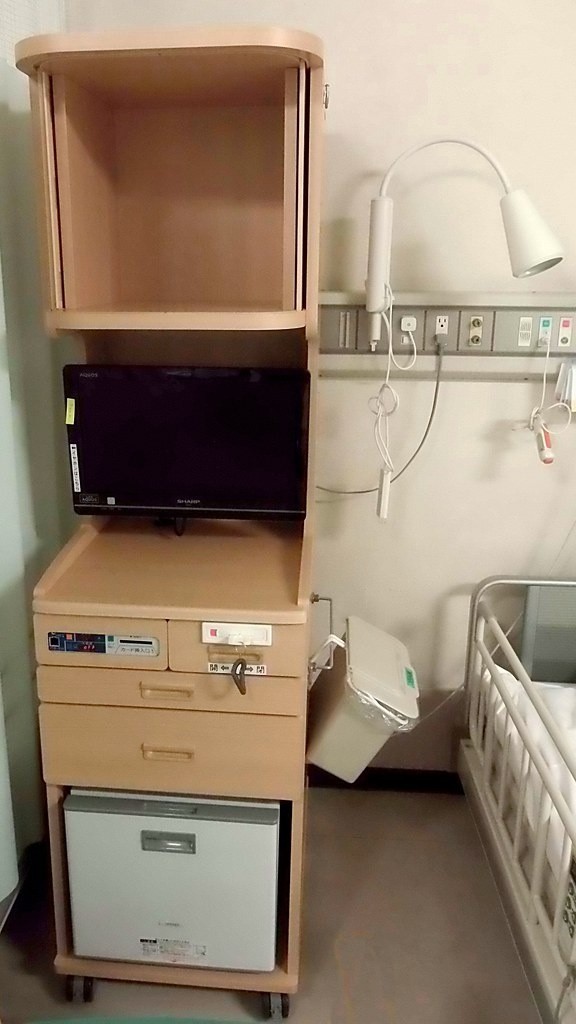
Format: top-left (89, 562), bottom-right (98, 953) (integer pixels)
top-left (365, 136), bottom-right (565, 352)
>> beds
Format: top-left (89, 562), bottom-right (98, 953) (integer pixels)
top-left (456, 574), bottom-right (576, 1024)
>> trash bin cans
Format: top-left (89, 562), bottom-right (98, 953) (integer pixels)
top-left (305, 614), bottom-right (421, 785)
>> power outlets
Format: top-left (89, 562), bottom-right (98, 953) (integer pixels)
top-left (434, 315), bottom-right (449, 346)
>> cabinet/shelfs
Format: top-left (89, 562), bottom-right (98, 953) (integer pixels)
top-left (0, 26), bottom-right (329, 1024)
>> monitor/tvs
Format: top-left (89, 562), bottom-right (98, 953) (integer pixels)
top-left (60, 364), bottom-right (309, 538)
